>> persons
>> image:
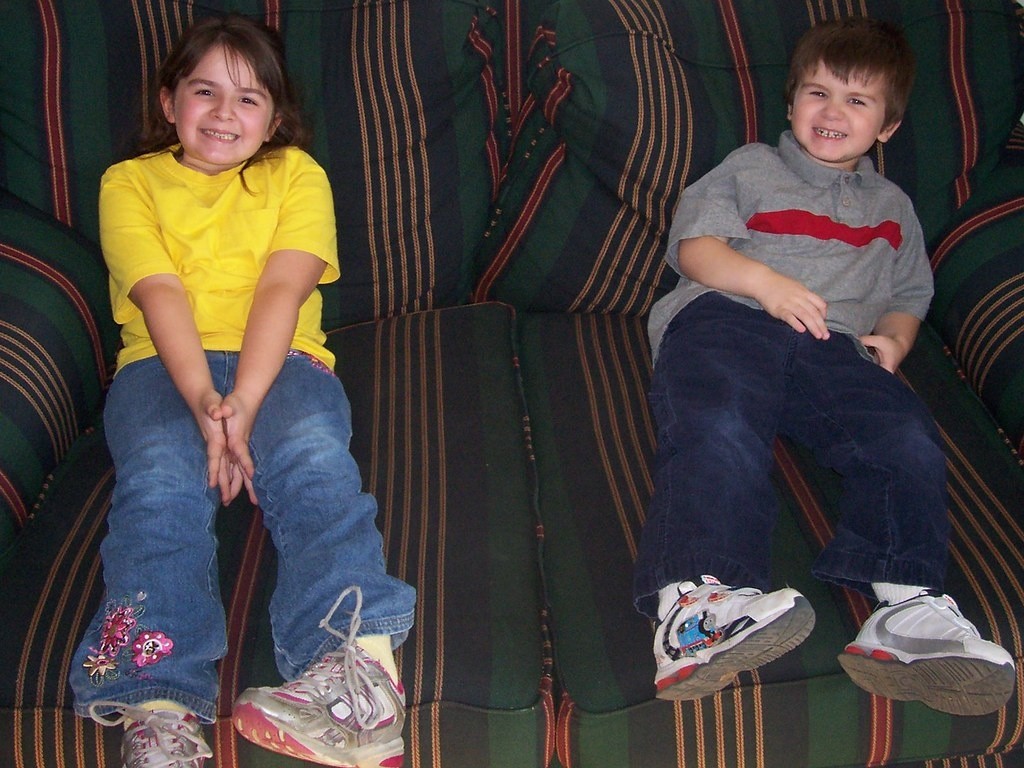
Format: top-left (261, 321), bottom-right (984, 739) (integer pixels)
top-left (69, 12), bottom-right (418, 767)
top-left (634, 16), bottom-right (1018, 717)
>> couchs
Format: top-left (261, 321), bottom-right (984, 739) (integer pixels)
top-left (0, 0), bottom-right (1024, 768)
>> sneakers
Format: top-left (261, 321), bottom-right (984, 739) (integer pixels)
top-left (232, 585), bottom-right (406, 768)
top-left (652, 573), bottom-right (813, 701)
top-left (836, 589), bottom-right (1013, 716)
top-left (89, 701), bottom-right (213, 768)
